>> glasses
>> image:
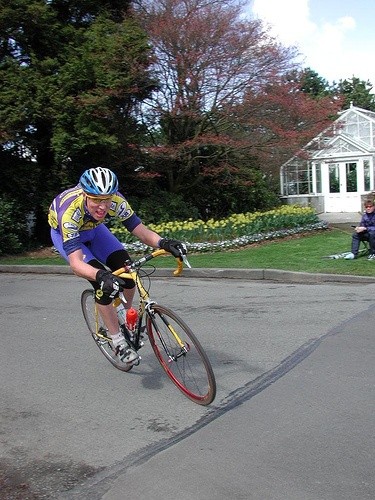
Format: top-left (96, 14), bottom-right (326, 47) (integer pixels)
top-left (83, 191), bottom-right (113, 203)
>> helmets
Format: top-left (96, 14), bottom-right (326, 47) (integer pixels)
top-left (80, 166), bottom-right (118, 195)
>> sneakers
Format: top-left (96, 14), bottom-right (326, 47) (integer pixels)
top-left (108, 337), bottom-right (142, 365)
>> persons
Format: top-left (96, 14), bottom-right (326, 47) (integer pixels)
top-left (344, 198), bottom-right (375, 260)
top-left (48, 167), bottom-right (187, 363)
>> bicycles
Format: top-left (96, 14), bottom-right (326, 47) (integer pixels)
top-left (80, 248), bottom-right (217, 407)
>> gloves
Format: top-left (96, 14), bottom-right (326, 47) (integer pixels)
top-left (159, 238), bottom-right (187, 261)
top-left (95, 269), bottom-right (126, 298)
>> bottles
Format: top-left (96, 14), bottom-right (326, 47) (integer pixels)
top-left (125, 307), bottom-right (137, 334)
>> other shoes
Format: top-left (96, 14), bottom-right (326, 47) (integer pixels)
top-left (343, 253), bottom-right (360, 260)
top-left (367, 254), bottom-right (375, 260)
